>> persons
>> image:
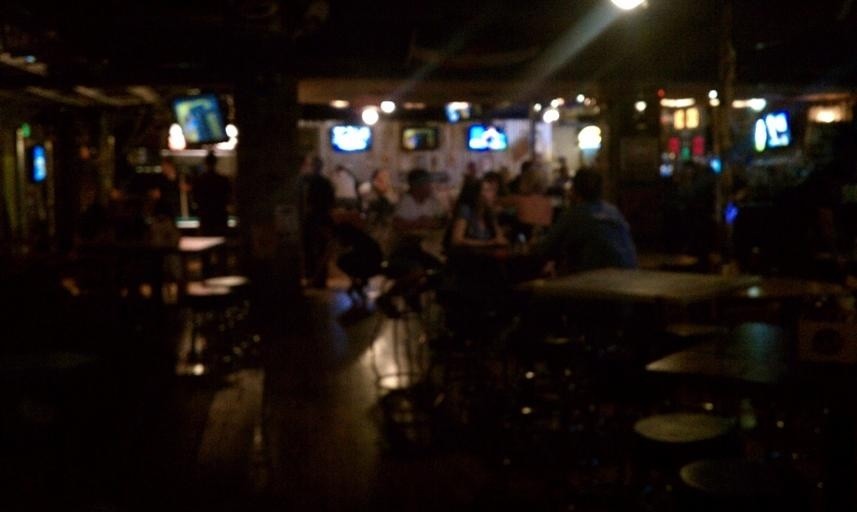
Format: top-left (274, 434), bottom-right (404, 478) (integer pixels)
top-left (650, 128), bottom-right (856, 257)
top-left (1, 139), bottom-right (568, 328)
top-left (542, 166), bottom-right (641, 276)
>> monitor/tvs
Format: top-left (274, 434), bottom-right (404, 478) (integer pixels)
top-left (466, 124), bottom-right (507, 151)
top-left (30, 144), bottom-right (48, 183)
top-left (170, 92), bottom-right (229, 148)
top-left (329, 124), bottom-right (372, 152)
top-left (764, 111), bottom-right (792, 150)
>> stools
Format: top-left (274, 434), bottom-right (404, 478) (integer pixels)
top-left (177, 234), bottom-right (855, 511)
